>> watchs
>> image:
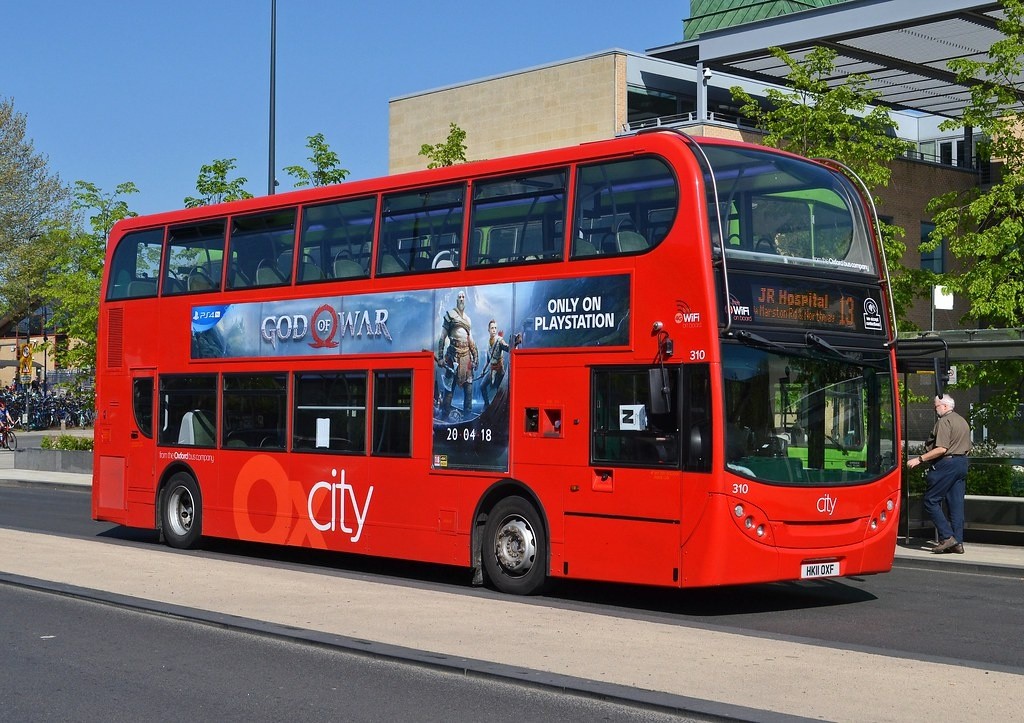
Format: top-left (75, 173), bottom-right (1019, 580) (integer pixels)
top-left (918, 456), bottom-right (924, 463)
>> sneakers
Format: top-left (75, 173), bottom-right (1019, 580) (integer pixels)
top-left (931, 536), bottom-right (960, 553)
top-left (943, 543), bottom-right (964, 553)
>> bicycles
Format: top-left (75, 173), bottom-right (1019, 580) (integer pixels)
top-left (0, 419), bottom-right (17, 451)
top-left (0, 380), bottom-right (93, 429)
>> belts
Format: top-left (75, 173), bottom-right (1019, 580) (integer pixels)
top-left (942, 455), bottom-right (953, 458)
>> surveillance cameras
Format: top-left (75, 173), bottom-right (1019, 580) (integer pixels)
top-left (704, 69), bottom-right (712, 79)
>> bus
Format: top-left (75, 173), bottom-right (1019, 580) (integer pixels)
top-left (92, 124), bottom-right (950, 597)
top-left (192, 185), bottom-right (869, 473)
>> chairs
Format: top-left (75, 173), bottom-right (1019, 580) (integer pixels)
top-left (223, 429), bottom-right (353, 453)
top-left (125, 214), bottom-right (780, 299)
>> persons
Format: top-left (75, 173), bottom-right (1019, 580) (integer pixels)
top-left (906, 394), bottom-right (972, 554)
top-left (0, 402), bottom-right (15, 447)
top-left (438, 291), bottom-right (522, 421)
top-left (32, 376), bottom-right (40, 391)
top-left (40, 380), bottom-right (44, 391)
